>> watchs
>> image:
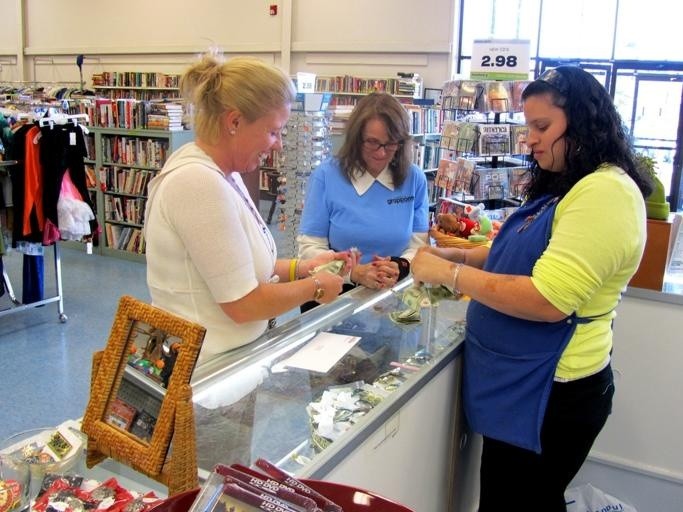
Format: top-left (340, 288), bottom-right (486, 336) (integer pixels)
top-left (310, 275), bottom-right (324, 300)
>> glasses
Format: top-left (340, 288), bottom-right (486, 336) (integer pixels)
top-left (360, 132), bottom-right (404, 153)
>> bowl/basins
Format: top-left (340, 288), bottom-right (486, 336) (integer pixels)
top-left (0, 428), bottom-right (84, 476)
top-left (0, 454), bottom-right (31, 512)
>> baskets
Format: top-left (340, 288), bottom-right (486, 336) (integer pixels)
top-left (428, 223), bottom-right (490, 249)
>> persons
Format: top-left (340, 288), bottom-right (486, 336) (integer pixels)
top-left (410, 67), bottom-right (650, 511)
top-left (143, 53), bottom-right (360, 476)
top-left (292, 91), bottom-right (429, 425)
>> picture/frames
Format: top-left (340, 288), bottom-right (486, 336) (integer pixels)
top-left (80, 292), bottom-right (207, 498)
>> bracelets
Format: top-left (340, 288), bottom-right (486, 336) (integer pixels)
top-left (451, 262), bottom-right (463, 295)
top-left (391, 256), bottom-right (409, 282)
top-left (289, 258), bottom-right (300, 282)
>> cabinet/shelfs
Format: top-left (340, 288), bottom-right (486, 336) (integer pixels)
top-left (60, 126), bottom-right (194, 263)
top-left (332, 131), bottom-right (437, 209)
